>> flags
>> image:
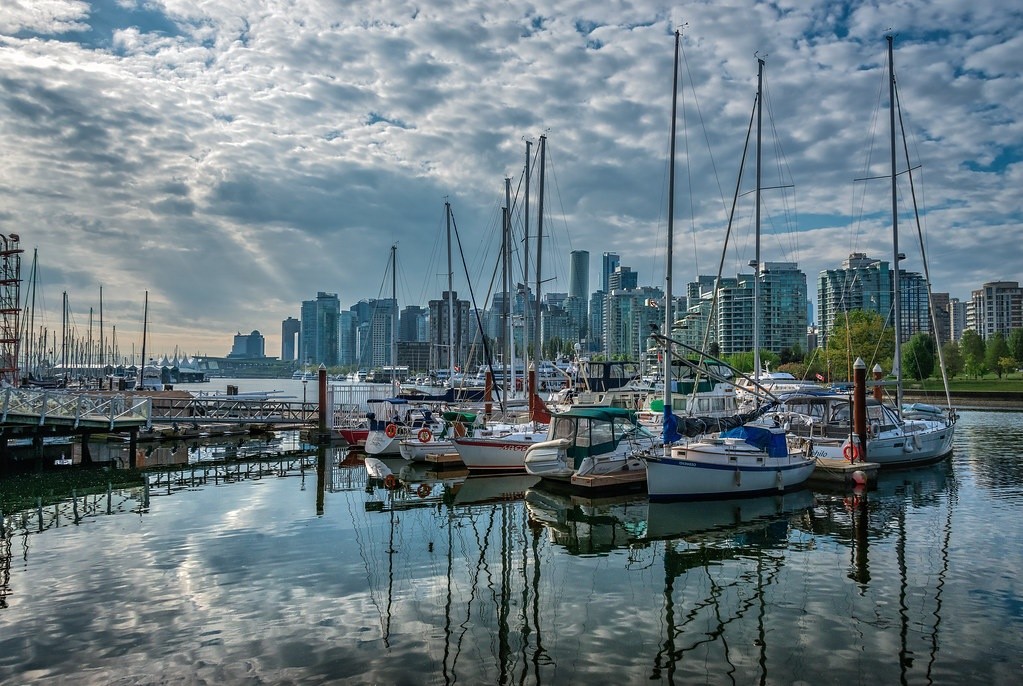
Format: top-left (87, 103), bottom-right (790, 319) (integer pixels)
top-left (532, 394), bottom-right (555, 424)
top-left (815, 373), bottom-right (824, 381)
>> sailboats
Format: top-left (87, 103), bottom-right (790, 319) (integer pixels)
top-left (336, 27), bottom-right (960, 498)
top-left (15, 246), bottom-right (162, 393)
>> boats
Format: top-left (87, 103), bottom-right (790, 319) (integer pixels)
top-left (339, 452), bottom-right (950, 685)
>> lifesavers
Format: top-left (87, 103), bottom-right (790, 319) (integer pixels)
top-left (385, 475), bottom-right (395, 489)
top-left (842, 444), bottom-right (857, 460)
top-left (385, 424), bottom-right (396, 438)
top-left (417, 429), bottom-right (431, 443)
top-left (417, 485), bottom-right (430, 497)
top-left (845, 496), bottom-right (858, 509)
